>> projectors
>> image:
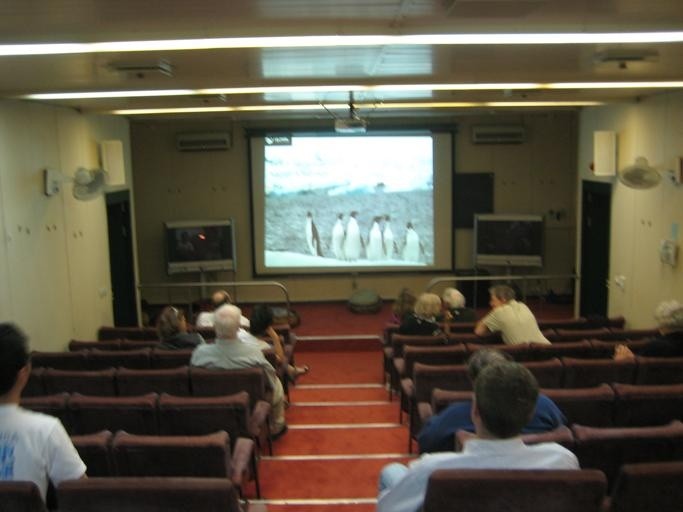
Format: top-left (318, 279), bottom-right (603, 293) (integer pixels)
top-left (335, 119), bottom-right (367, 133)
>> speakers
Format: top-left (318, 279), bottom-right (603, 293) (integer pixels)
top-left (97, 140), bottom-right (125, 185)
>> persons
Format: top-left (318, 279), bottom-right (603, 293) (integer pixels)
top-left (245, 305), bottom-right (310, 386)
top-left (191, 304), bottom-right (287, 442)
top-left (611, 300), bottom-right (683, 360)
top-left (376, 360), bottom-right (580, 512)
top-left (381, 287), bottom-right (475, 333)
top-left (0, 321), bottom-right (87, 502)
top-left (149, 305), bottom-right (207, 350)
top-left (474, 283), bottom-right (551, 345)
top-left (196, 290), bottom-right (250, 330)
top-left (417, 347), bottom-right (565, 452)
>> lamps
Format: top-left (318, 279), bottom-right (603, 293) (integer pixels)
top-left (101, 140), bottom-right (125, 186)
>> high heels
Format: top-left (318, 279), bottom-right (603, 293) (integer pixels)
top-left (289, 365), bottom-right (310, 386)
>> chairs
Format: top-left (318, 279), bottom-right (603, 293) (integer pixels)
top-left (419, 469), bottom-right (607, 512)
top-left (609, 463), bottom-right (683, 512)
top-left (381, 317), bottom-right (683, 468)
top-left (1, 326), bottom-right (293, 512)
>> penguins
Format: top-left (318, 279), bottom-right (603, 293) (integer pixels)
top-left (382, 215), bottom-right (398, 258)
top-left (342, 211), bottom-right (364, 259)
top-left (365, 215), bottom-right (383, 259)
top-left (402, 222), bottom-right (425, 263)
top-left (330, 212), bottom-right (345, 258)
top-left (304, 211), bottom-right (323, 257)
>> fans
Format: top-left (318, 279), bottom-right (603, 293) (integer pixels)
top-left (618, 157), bottom-right (681, 190)
top-left (45, 167), bottom-right (109, 202)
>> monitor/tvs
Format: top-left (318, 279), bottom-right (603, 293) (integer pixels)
top-left (474, 214), bottom-right (545, 268)
top-left (164, 217), bottom-right (237, 274)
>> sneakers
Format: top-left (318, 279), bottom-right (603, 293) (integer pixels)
top-left (268, 424), bottom-right (288, 443)
